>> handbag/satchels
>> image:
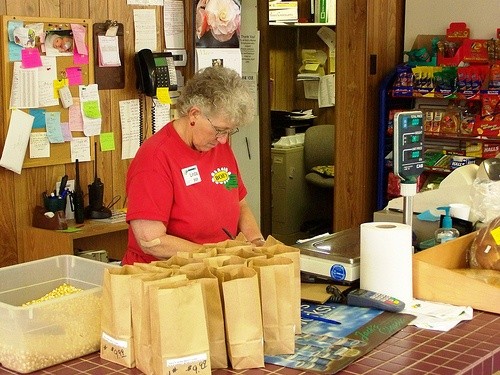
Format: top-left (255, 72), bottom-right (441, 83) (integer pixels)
top-left (99, 232), bottom-right (302, 375)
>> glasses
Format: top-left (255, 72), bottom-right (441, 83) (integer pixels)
top-left (203, 112), bottom-right (240, 138)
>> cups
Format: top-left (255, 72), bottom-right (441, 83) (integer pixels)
top-left (44, 196), bottom-right (67, 214)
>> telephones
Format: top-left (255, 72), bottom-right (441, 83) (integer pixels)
top-left (133, 49), bottom-right (178, 96)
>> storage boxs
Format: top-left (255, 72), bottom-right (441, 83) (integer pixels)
top-left (411, 231), bottom-right (500, 315)
top-left (0, 254), bottom-right (119, 374)
top-left (388, 22), bottom-right (500, 104)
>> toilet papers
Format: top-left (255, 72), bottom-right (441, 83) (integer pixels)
top-left (359, 221), bottom-right (413, 306)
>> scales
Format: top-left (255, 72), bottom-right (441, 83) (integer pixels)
top-left (291, 110), bottom-right (426, 284)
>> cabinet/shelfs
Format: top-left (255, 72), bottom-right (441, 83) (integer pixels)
top-left (376, 69), bottom-right (500, 210)
top-left (271, 145), bottom-right (327, 245)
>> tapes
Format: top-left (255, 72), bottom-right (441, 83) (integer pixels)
top-left (44, 211), bottom-right (55, 218)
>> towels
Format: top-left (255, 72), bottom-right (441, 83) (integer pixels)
top-left (317, 74), bottom-right (335, 109)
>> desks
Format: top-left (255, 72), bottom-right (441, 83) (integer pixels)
top-left (23, 207), bottom-right (129, 261)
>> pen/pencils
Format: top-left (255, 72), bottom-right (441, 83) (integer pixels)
top-left (42, 192), bottom-right (48, 198)
top-left (61, 188), bottom-right (69, 199)
top-left (222, 227), bottom-right (234, 240)
top-left (301, 312), bottom-right (341, 324)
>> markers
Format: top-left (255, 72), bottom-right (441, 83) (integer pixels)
top-left (58, 175), bottom-right (68, 195)
top-left (51, 192), bottom-right (55, 199)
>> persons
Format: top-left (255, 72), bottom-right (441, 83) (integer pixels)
top-left (50, 33), bottom-right (74, 53)
top-left (118, 66), bottom-right (264, 266)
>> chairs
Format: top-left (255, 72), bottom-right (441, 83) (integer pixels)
top-left (301, 125), bottom-right (334, 235)
